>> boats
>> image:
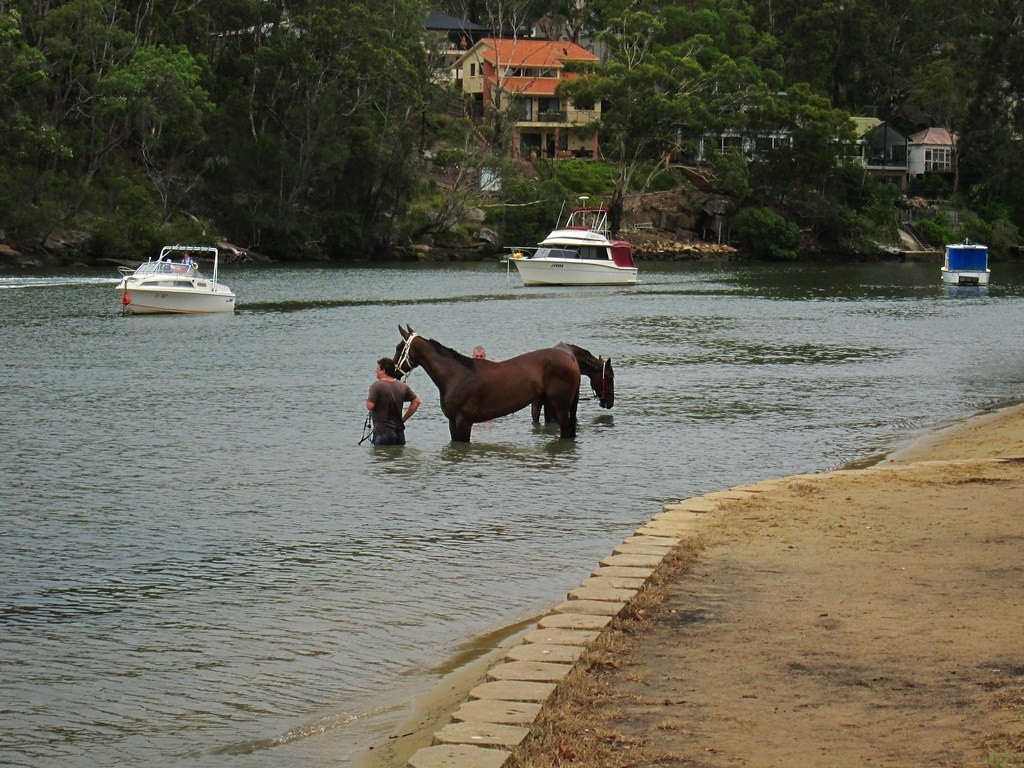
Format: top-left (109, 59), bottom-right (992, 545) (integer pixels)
top-left (113, 244), bottom-right (236, 315)
top-left (501, 196), bottom-right (638, 288)
top-left (941, 238), bottom-right (991, 285)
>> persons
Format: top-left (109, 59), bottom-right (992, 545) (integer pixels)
top-left (181, 250), bottom-right (194, 267)
top-left (162, 259), bottom-right (179, 275)
top-left (366, 357), bottom-right (421, 447)
top-left (472, 345), bottom-right (487, 360)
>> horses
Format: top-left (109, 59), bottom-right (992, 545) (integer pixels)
top-left (390, 322), bottom-right (582, 444)
top-left (530, 339), bottom-right (615, 424)
